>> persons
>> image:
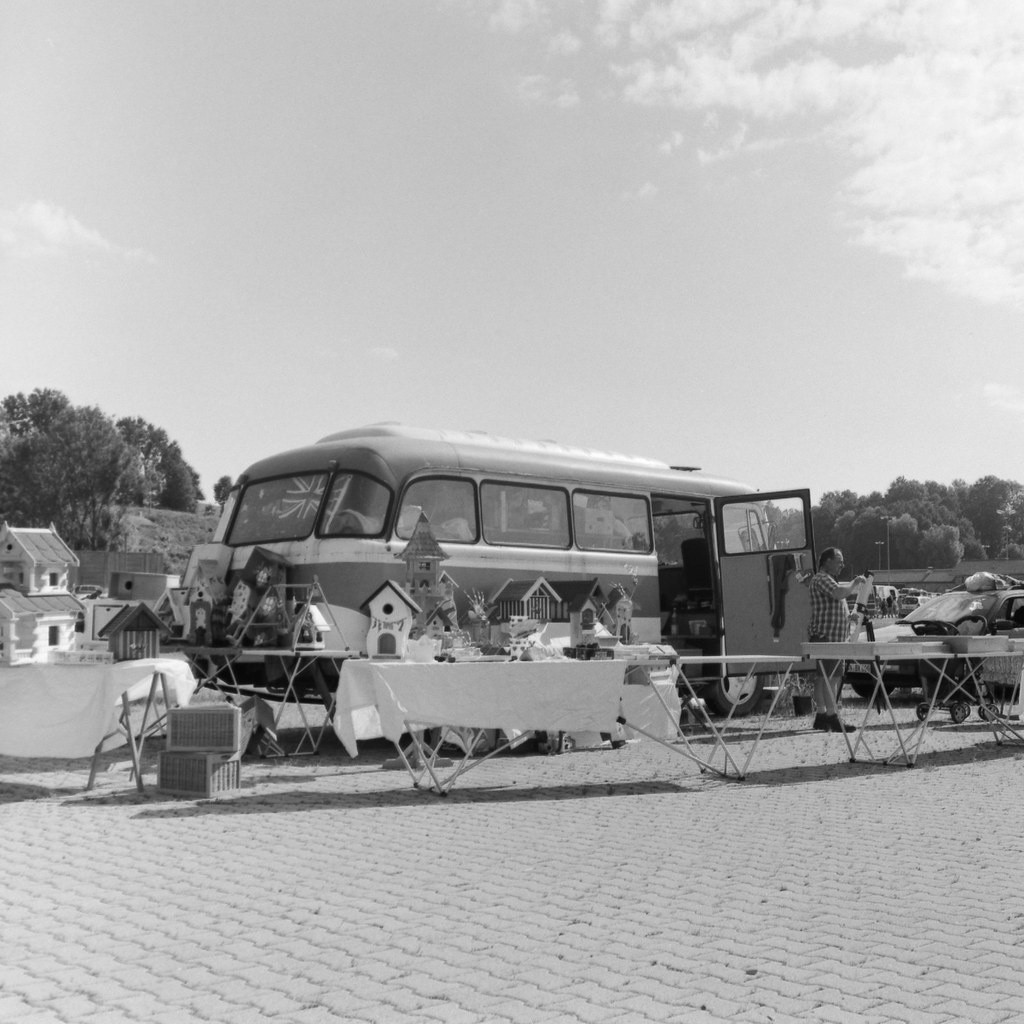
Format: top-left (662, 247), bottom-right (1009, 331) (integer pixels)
top-left (879, 587), bottom-right (899, 619)
top-left (809, 546), bottom-right (868, 733)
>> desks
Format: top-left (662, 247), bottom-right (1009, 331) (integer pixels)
top-left (809, 651), bottom-right (1024, 772)
top-left (182, 647), bottom-right (353, 759)
top-left (333, 653), bottom-right (801, 784)
top-left (0, 657), bottom-right (197, 791)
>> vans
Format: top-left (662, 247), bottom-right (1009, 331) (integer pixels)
top-left (875, 585), bottom-right (899, 601)
top-left (899, 596), bottom-right (930, 617)
top-left (837, 582), bottom-right (877, 619)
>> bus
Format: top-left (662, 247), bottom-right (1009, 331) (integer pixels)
top-left (180, 420), bottom-right (818, 715)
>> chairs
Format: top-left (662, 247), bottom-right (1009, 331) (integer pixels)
top-left (680, 538), bottom-right (709, 595)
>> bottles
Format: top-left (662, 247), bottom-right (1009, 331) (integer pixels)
top-left (670, 607), bottom-right (680, 635)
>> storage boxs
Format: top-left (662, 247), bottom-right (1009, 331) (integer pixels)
top-left (814, 635), bottom-right (1024, 655)
top-left (155, 705), bottom-right (241, 799)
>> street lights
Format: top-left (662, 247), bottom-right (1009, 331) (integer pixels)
top-left (875, 541), bottom-right (885, 571)
top-left (996, 509), bottom-right (1016, 577)
top-left (880, 515), bottom-right (894, 586)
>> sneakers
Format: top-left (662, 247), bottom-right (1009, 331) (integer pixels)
top-left (814, 713), bottom-right (856, 733)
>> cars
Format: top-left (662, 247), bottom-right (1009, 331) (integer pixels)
top-left (844, 585), bottom-right (1024, 699)
top-left (899, 588), bottom-right (938, 601)
